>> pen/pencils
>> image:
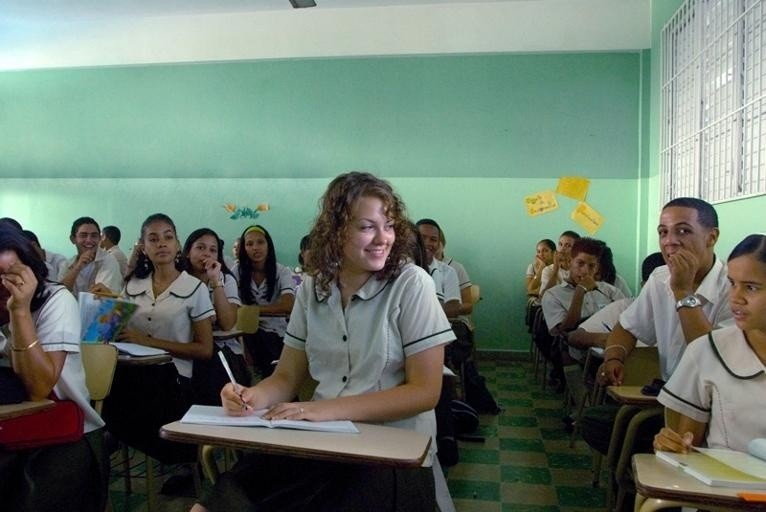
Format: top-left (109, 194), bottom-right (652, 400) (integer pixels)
top-left (595, 288), bottom-right (605, 295)
top-left (601, 321), bottom-right (611, 331)
top-left (217, 350), bottom-right (247, 410)
top-left (93, 292), bottom-right (123, 297)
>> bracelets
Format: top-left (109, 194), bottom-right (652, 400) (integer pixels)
top-left (607, 357), bottom-right (624, 365)
top-left (605, 345), bottom-right (627, 360)
top-left (10, 339), bottom-right (39, 352)
top-left (534, 276), bottom-right (541, 282)
top-left (99, 214), bottom-right (216, 497)
top-left (577, 285), bottom-right (588, 294)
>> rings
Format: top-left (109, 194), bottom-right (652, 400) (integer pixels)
top-left (299, 408), bottom-right (304, 413)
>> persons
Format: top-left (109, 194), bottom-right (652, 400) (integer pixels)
top-left (541, 237), bottom-right (625, 385)
top-left (591, 239), bottom-right (632, 300)
top-left (0, 221), bottom-right (106, 512)
top-left (99, 226), bottom-right (128, 282)
top-left (23, 229), bottom-right (68, 286)
top-left (180, 226), bottom-right (251, 410)
top-left (578, 198), bottom-right (741, 472)
top-left (57, 217), bottom-right (122, 300)
top-left (189, 171), bottom-right (457, 512)
top-left (291, 235), bottom-right (320, 295)
top-left (396, 220), bottom-right (428, 271)
top-left (568, 252), bottom-right (671, 353)
top-left (526, 239), bottom-right (556, 294)
top-left (415, 218), bottom-right (463, 432)
top-left (0, 217), bottom-right (55, 285)
top-left (652, 236), bottom-right (766, 456)
top-left (227, 226), bottom-right (295, 383)
top-left (434, 230), bottom-right (473, 391)
top-left (232, 237), bottom-right (242, 261)
top-left (538, 231), bottom-right (582, 300)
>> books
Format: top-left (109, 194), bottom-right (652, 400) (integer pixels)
top-left (109, 342), bottom-right (169, 357)
top-left (79, 292), bottom-right (140, 344)
top-left (181, 403), bottom-right (360, 434)
top-left (655, 449), bottom-right (765, 489)
top-left (694, 437), bottom-right (766, 477)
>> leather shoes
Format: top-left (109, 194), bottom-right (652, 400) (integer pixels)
top-left (440, 439), bottom-right (459, 464)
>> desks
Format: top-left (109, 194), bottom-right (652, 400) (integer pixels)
top-left (118, 355), bottom-right (216, 509)
top-left (0, 399), bottom-right (56, 421)
top-left (631, 453), bottom-right (766, 512)
top-left (158, 420), bottom-right (432, 485)
top-left (606, 386), bottom-right (664, 512)
top-left (213, 330), bottom-right (244, 340)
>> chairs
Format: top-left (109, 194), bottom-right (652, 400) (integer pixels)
top-left (79, 343), bottom-right (118, 417)
top-left (593, 347), bottom-right (661, 488)
top-left (237, 305), bottom-right (260, 333)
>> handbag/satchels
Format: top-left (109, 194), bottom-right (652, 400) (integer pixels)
top-left (464, 360), bottom-right (501, 415)
top-left (452, 398), bottom-right (479, 430)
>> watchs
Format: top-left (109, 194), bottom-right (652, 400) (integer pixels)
top-left (675, 295), bottom-right (702, 312)
top-left (209, 279), bottom-right (225, 288)
